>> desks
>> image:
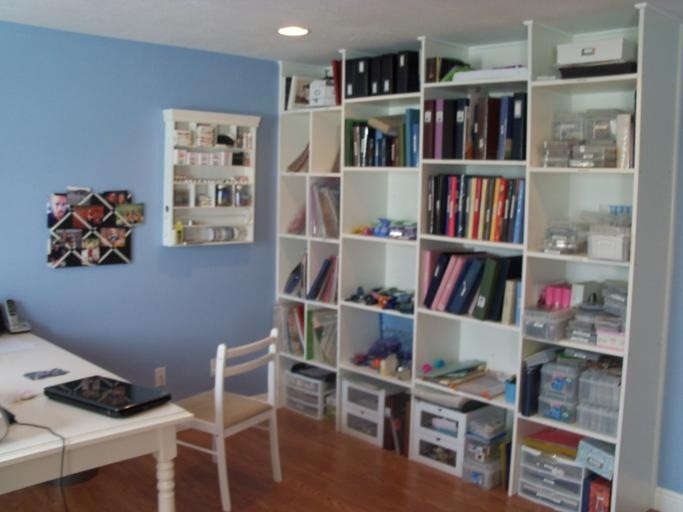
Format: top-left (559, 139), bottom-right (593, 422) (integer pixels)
top-left (0, 327), bottom-right (196, 512)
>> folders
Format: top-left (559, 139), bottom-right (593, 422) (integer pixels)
top-left (346, 51), bottom-right (419, 98)
top-left (424, 91), bottom-right (526, 160)
top-left (420, 249), bottom-right (522, 328)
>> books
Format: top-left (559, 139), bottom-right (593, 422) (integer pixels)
top-left (419, 174), bottom-right (525, 326)
top-left (344, 107), bottom-right (418, 167)
top-left (281, 145), bottom-right (342, 304)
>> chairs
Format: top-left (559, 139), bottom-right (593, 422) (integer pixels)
top-left (177, 327), bottom-right (282, 512)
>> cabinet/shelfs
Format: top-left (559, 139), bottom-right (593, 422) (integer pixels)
top-left (162, 109), bottom-right (261, 249)
top-left (273, 2), bottom-right (683, 512)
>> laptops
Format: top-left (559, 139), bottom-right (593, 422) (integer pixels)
top-left (44, 376), bottom-right (171, 418)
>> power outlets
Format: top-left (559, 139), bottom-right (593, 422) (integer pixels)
top-left (155, 367), bottom-right (167, 388)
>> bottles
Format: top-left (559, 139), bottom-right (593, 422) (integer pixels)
top-left (217, 186), bottom-right (250, 206)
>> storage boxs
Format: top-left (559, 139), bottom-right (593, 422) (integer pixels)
top-left (585, 215), bottom-right (631, 263)
top-left (284, 369), bottom-right (335, 422)
top-left (525, 306), bottom-right (575, 341)
top-left (518, 362), bottom-right (619, 512)
top-left (556, 39), bottom-right (636, 75)
top-left (411, 398), bottom-right (508, 490)
top-left (341, 376), bottom-right (406, 449)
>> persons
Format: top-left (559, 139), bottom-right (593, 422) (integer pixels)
top-left (45, 192), bottom-right (142, 267)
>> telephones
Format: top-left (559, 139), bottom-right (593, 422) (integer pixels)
top-left (4, 299), bottom-right (32, 334)
